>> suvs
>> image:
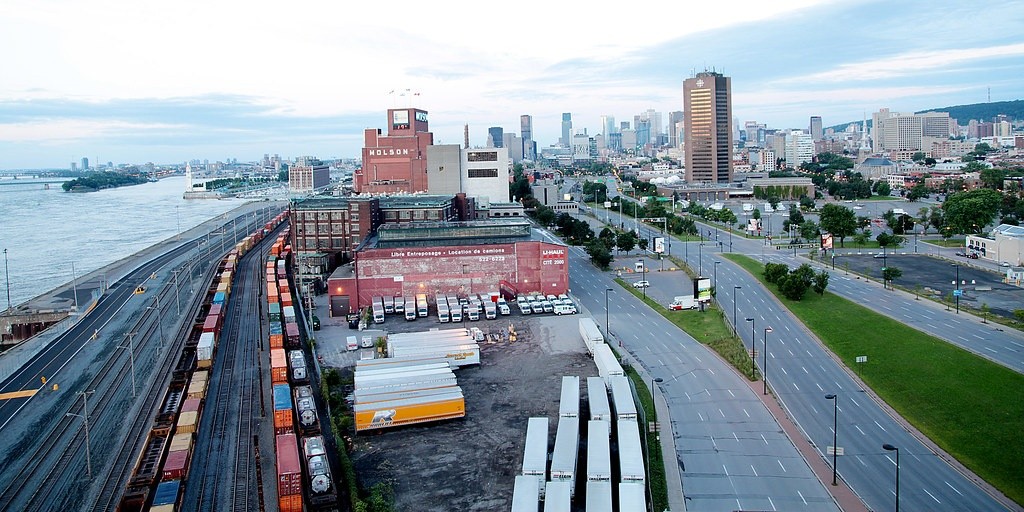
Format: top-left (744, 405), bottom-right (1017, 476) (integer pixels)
top-left (633, 281), bottom-right (649, 288)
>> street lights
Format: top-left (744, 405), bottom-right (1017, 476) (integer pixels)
top-left (826, 395), bottom-right (837, 486)
top-left (116, 333), bottom-right (140, 399)
top-left (952, 264), bottom-right (962, 314)
top-left (883, 444), bottom-right (900, 512)
top-left (714, 261), bottom-right (720, 296)
top-left (745, 318), bottom-right (755, 380)
top-left (606, 288), bottom-right (614, 337)
top-left (733, 287), bottom-right (741, 334)
top-left (65, 390), bottom-right (98, 479)
top-left (150, 204), bottom-right (286, 346)
top-left (764, 329), bottom-right (772, 394)
top-left (4, 248), bottom-right (11, 309)
top-left (938, 227), bottom-right (951, 257)
top-left (638, 260), bottom-right (646, 299)
top-left (699, 243), bottom-right (706, 276)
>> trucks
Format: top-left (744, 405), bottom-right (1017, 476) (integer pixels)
top-left (517, 294), bottom-right (577, 316)
top-left (346, 336), bottom-right (359, 351)
top-left (669, 294), bottom-right (699, 311)
top-left (371, 293), bottom-right (510, 323)
top-left (361, 336), bottom-right (374, 347)
